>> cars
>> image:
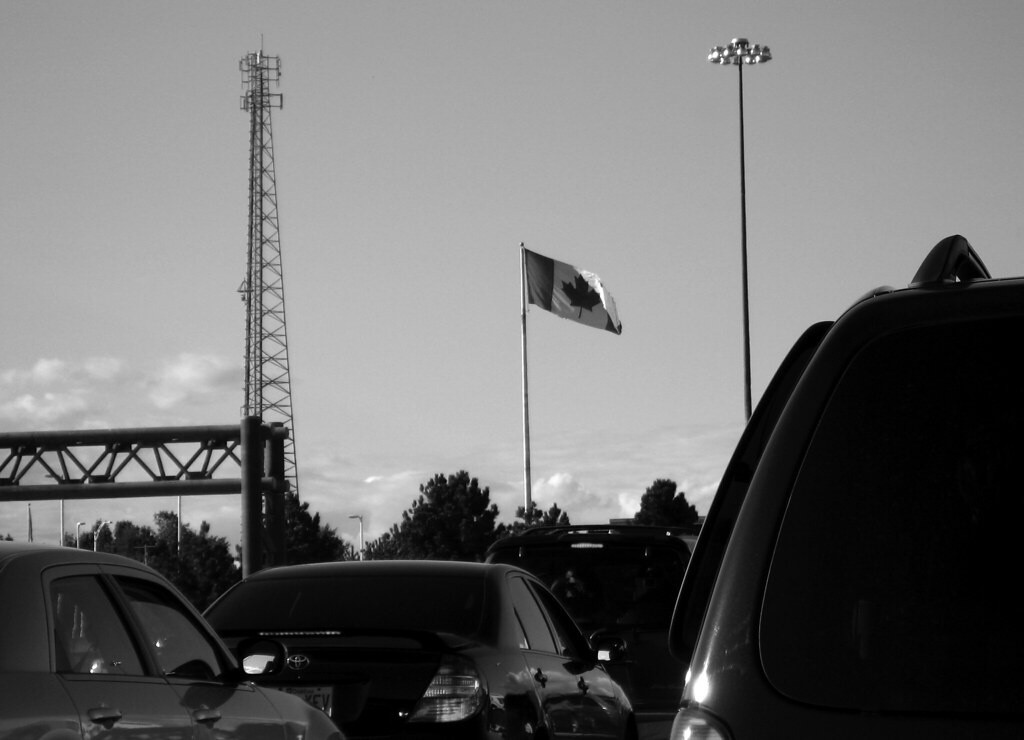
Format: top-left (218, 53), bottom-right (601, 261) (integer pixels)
top-left (198, 560), bottom-right (640, 740)
top-left (0, 539), bottom-right (350, 740)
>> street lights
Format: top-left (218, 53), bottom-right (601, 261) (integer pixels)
top-left (705, 36), bottom-right (773, 427)
top-left (94, 520), bottom-right (112, 552)
top-left (349, 513), bottom-right (365, 559)
top-left (76, 522), bottom-right (86, 549)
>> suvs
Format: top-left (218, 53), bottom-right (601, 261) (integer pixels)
top-left (661, 231), bottom-right (1023, 740)
top-left (483, 520), bottom-right (703, 740)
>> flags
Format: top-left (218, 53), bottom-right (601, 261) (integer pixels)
top-left (520, 249), bottom-right (622, 335)
